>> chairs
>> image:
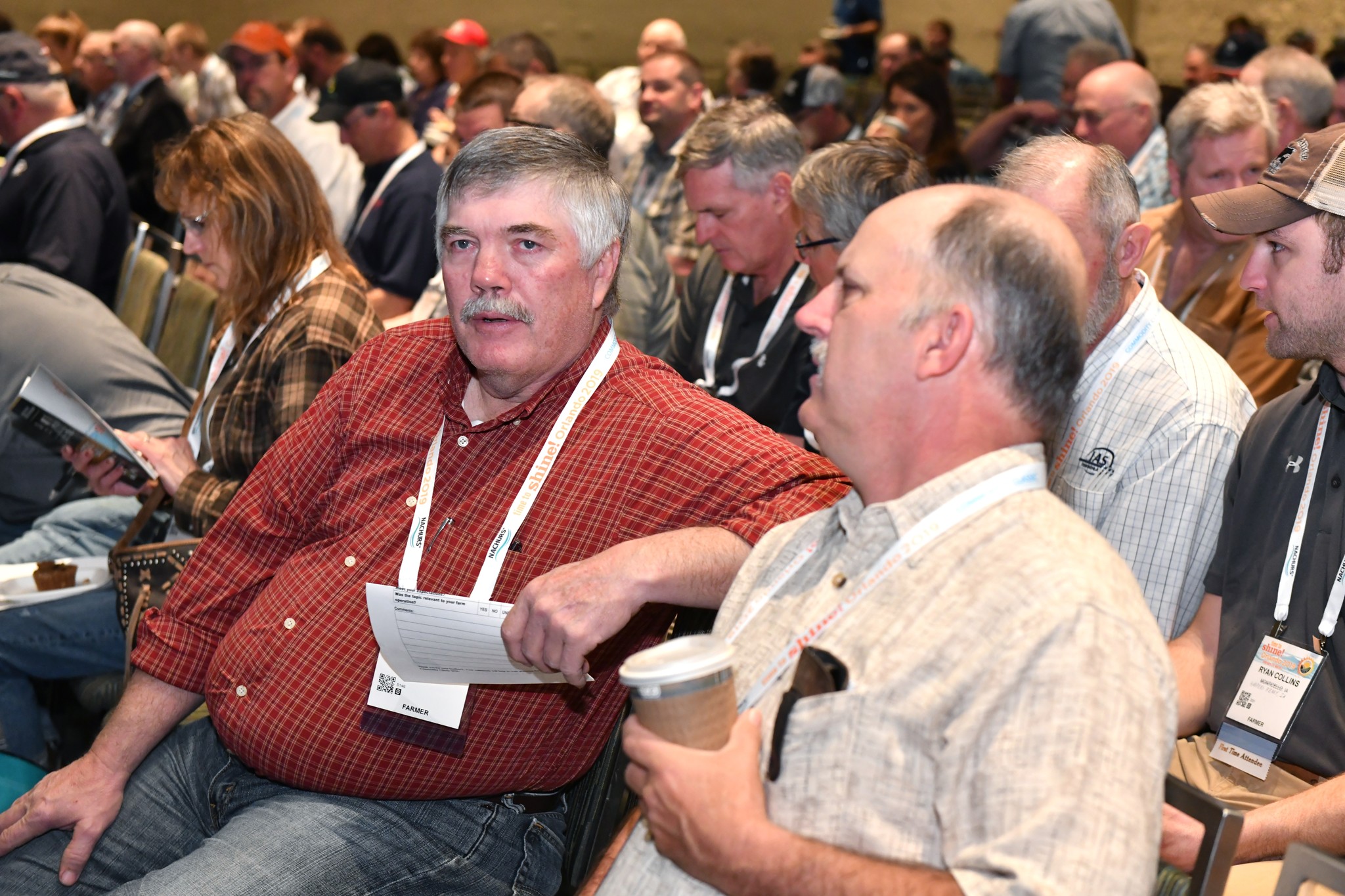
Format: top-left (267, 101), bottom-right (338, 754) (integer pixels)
top-left (114, 213), bottom-right (222, 390)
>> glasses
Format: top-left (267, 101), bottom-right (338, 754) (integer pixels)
top-left (795, 229), bottom-right (840, 260)
top-left (503, 118), bottom-right (554, 131)
top-left (182, 202), bottom-right (217, 238)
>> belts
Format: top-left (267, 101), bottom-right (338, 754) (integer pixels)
top-left (480, 785), bottom-right (567, 814)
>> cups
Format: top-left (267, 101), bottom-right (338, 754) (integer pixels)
top-left (618, 633), bottom-right (738, 751)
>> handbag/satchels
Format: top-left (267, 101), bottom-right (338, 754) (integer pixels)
top-left (111, 537), bottom-right (204, 658)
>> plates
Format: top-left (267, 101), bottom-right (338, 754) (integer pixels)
top-left (0, 567), bottom-right (111, 603)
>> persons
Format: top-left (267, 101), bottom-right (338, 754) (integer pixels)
top-left (1137, 82), bottom-right (1306, 409)
top-left (574, 183), bottom-right (1173, 896)
top-left (0, 8), bottom-right (363, 311)
top-left (660, 96), bottom-right (932, 453)
top-left (1161, 120), bottom-right (1345, 896)
top-left (0, 124), bottom-right (848, 896)
top-left (993, 0), bottom-right (1134, 135)
top-left (961, 41), bottom-right (1119, 175)
top-left (1074, 60), bottom-right (1175, 212)
top-left (1240, 45), bottom-right (1337, 161)
top-left (383, 75), bottom-right (520, 330)
top-left (1, 113), bottom-right (383, 768)
top-left (281, 16), bottom-right (715, 291)
top-left (722, 0), bottom-right (972, 182)
top-left (508, 74), bottom-right (681, 359)
top-left (0, 263), bottom-right (195, 540)
top-left (309, 60), bottom-right (444, 321)
top-left (999, 134), bottom-right (1258, 639)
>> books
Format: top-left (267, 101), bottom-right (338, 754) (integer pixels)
top-left (0, 364), bottom-right (158, 489)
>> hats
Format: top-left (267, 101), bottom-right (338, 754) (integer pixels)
top-left (0, 32), bottom-right (64, 82)
top-left (441, 18), bottom-right (488, 48)
top-left (1190, 123), bottom-right (1345, 235)
top-left (220, 21), bottom-right (294, 60)
top-left (311, 59), bottom-right (401, 122)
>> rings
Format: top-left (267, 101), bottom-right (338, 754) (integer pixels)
top-left (145, 435), bottom-right (151, 442)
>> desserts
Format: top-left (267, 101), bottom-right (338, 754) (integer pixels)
top-left (34, 561), bottom-right (77, 590)
